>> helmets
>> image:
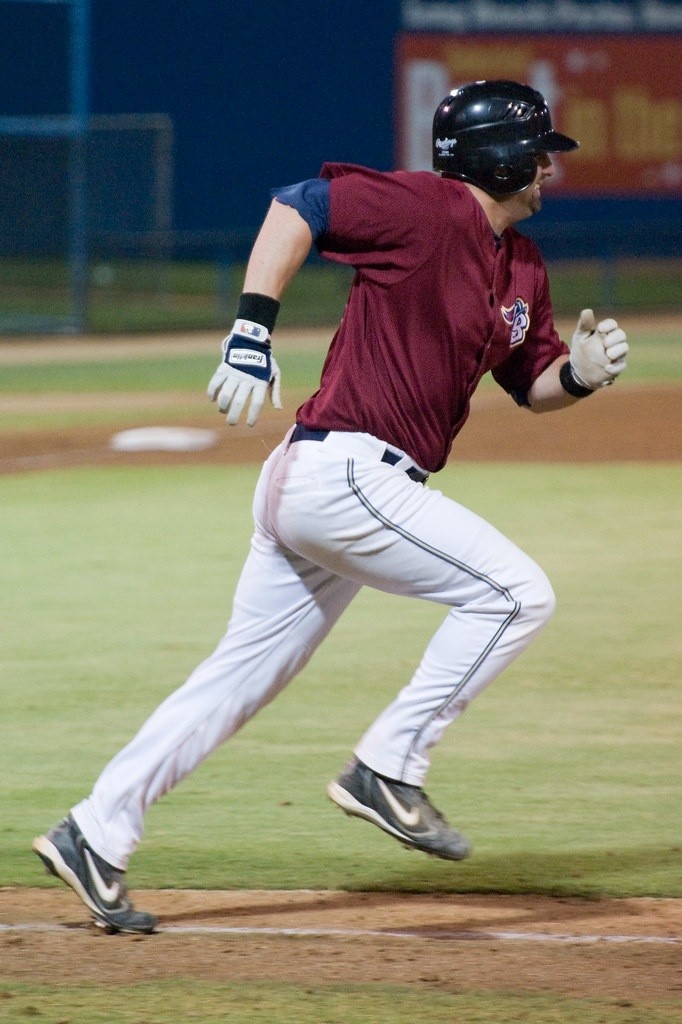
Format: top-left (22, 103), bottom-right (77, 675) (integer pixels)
top-left (433, 79), bottom-right (580, 195)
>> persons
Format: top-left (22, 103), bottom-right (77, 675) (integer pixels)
top-left (31, 80), bottom-right (629, 936)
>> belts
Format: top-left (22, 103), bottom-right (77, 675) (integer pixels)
top-left (289, 424), bottom-right (430, 484)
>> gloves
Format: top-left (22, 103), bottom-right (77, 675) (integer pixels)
top-left (559, 307), bottom-right (630, 400)
top-left (207, 292), bottom-right (283, 426)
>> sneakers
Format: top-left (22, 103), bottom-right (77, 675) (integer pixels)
top-left (328, 756), bottom-right (472, 861)
top-left (31, 811), bottom-right (156, 933)
